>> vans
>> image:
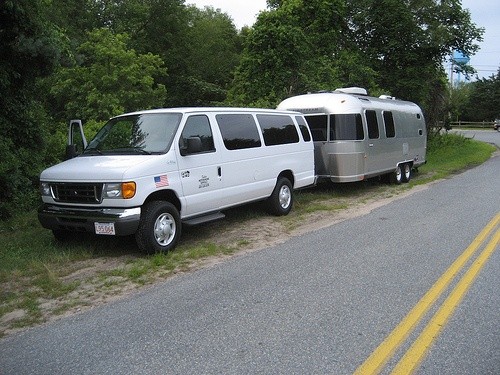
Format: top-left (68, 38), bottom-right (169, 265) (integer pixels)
top-left (37, 107), bottom-right (315, 252)
